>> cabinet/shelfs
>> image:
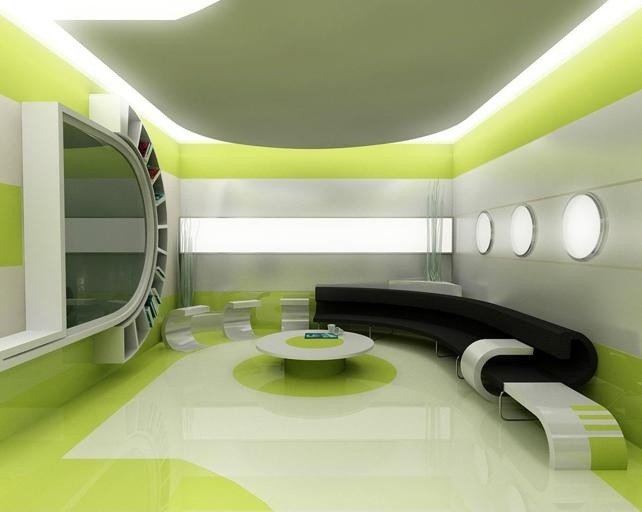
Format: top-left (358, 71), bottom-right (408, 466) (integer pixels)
top-left (88, 93), bottom-right (169, 363)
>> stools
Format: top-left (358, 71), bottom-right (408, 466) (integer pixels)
top-left (160, 298), bottom-right (309, 353)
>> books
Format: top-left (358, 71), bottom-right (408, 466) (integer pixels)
top-left (146, 267), bottom-right (168, 327)
top-left (139, 140), bottom-right (164, 200)
top-left (305, 332), bottom-right (339, 339)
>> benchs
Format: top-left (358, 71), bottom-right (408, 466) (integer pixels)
top-left (313, 286), bottom-right (598, 395)
top-left (498, 382), bottom-right (628, 470)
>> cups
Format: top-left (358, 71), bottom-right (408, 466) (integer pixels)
top-left (328, 324), bottom-right (344, 337)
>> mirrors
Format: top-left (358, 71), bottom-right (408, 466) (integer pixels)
top-left (20, 102), bottom-right (155, 338)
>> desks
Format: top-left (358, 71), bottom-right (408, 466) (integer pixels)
top-left (255, 329), bottom-right (373, 378)
